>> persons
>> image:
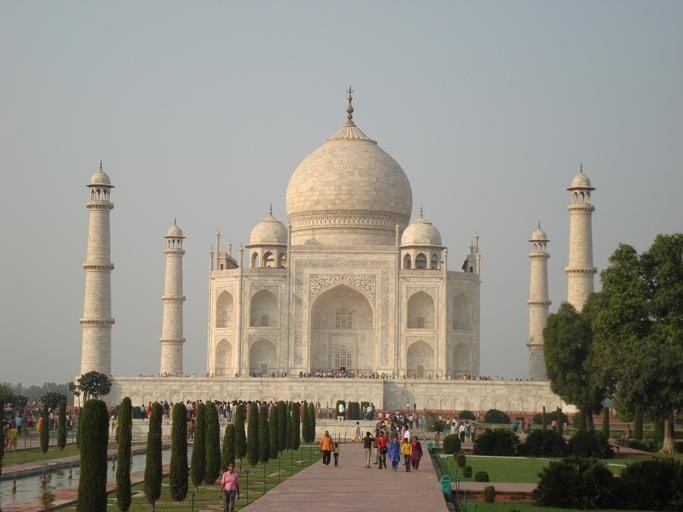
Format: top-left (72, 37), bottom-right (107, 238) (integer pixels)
top-left (218, 461), bottom-right (240, 512)
top-left (2, 400), bottom-right (119, 454)
top-left (141, 398), bottom-right (346, 438)
top-left (510, 418), bottom-right (518, 432)
top-left (563, 419), bottom-right (568, 433)
top-left (520, 418), bottom-right (524, 431)
top-left (235, 370), bottom-right (287, 378)
top-left (358, 371), bottom-right (395, 379)
top-left (352, 402), bottom-right (476, 472)
top-left (403, 373), bottom-right (439, 380)
top-left (551, 419), bottom-right (558, 431)
top-left (138, 371), bottom-right (191, 377)
top-left (320, 430), bottom-right (335, 468)
top-left (457, 374), bottom-right (491, 381)
top-left (614, 437), bottom-right (622, 453)
top-left (333, 443), bottom-right (339, 466)
top-left (527, 417), bottom-right (532, 430)
top-left (299, 369), bottom-right (355, 378)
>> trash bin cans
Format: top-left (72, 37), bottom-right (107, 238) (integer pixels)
top-left (512, 421), bottom-right (517, 432)
top-left (440, 474), bottom-right (453, 501)
top-left (187, 441), bottom-right (194, 468)
top-left (427, 440), bottom-right (433, 453)
